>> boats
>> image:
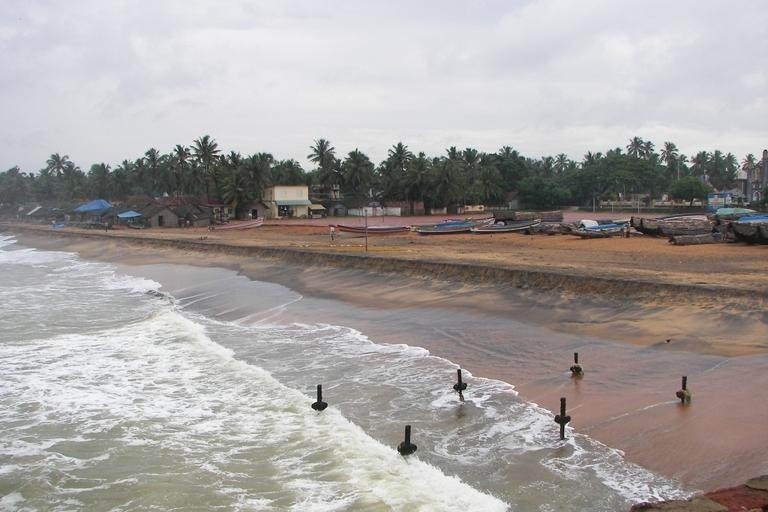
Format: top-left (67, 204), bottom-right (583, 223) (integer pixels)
top-left (211, 216), bottom-right (265, 231)
top-left (411, 209), bottom-right (563, 234)
top-left (129, 221), bottom-right (144, 229)
top-left (337, 224), bottom-right (411, 235)
top-left (541, 207), bottom-right (768, 245)
